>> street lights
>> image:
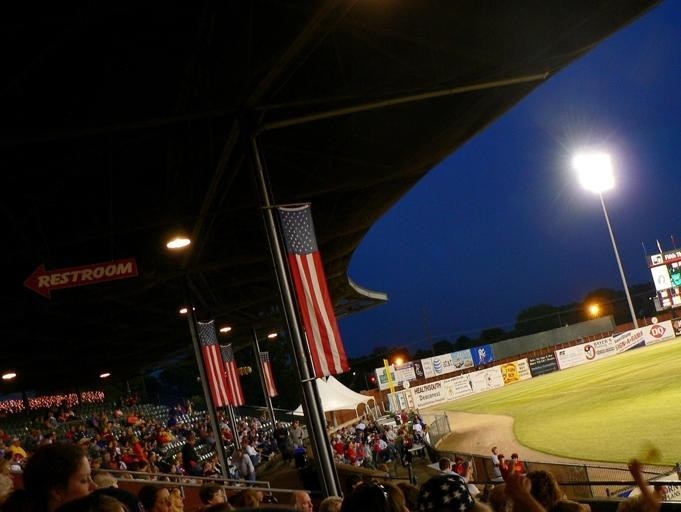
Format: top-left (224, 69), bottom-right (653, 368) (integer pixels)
top-left (166, 235), bottom-right (278, 487)
top-left (570, 150), bottom-right (640, 330)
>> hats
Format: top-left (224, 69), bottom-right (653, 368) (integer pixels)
top-left (417, 474), bottom-right (474, 512)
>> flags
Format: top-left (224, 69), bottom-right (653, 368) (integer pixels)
top-left (220, 340), bottom-right (247, 408)
top-left (276, 205), bottom-right (351, 380)
top-left (196, 319), bottom-right (233, 411)
top-left (259, 350), bottom-right (279, 400)
